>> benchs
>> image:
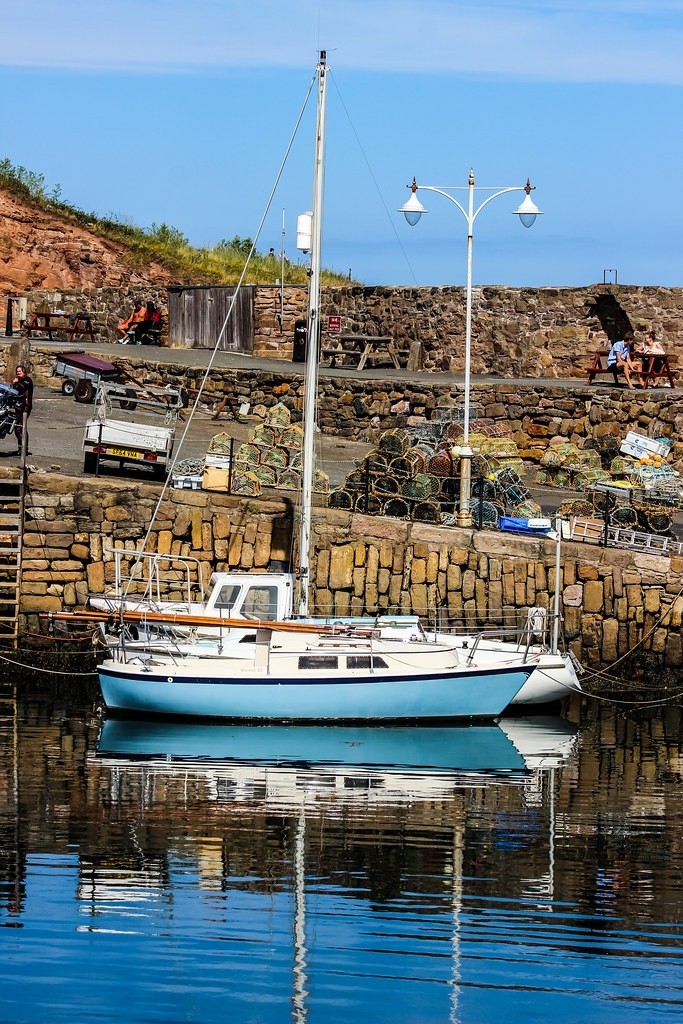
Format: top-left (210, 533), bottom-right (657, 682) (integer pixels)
top-left (375, 346), bottom-right (409, 353)
top-left (584, 367), bottom-right (656, 378)
top-left (24, 324), bottom-right (70, 333)
top-left (320, 348), bottom-right (361, 356)
top-left (75, 328), bottom-right (99, 335)
top-left (115, 319), bottom-right (161, 345)
top-left (661, 369), bottom-right (680, 374)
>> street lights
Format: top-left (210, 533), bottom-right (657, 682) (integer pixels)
top-left (394, 168), bottom-right (545, 532)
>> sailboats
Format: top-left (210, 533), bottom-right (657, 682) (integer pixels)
top-left (94, 46), bottom-right (596, 721)
top-left (93, 705), bottom-right (581, 1024)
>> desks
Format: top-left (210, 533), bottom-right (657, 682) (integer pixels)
top-left (26, 312), bottom-right (96, 343)
top-left (586, 349), bottom-right (675, 390)
top-left (329, 335), bottom-right (401, 372)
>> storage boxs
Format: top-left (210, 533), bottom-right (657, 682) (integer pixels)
top-left (619, 440), bottom-right (655, 460)
top-left (626, 430), bottom-right (671, 459)
top-left (205, 454), bottom-right (233, 470)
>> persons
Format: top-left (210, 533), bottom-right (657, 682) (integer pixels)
top-left (269, 248), bottom-right (288, 260)
top-left (607, 331), bottom-right (645, 390)
top-left (11, 365), bottom-right (34, 456)
top-left (636, 331), bottom-right (666, 388)
top-left (117, 301), bottom-right (146, 345)
top-left (135, 301), bottom-right (161, 345)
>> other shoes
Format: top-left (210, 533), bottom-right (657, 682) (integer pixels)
top-left (135, 341), bottom-right (142, 345)
top-left (628, 384), bottom-right (635, 390)
top-left (640, 385), bottom-right (652, 389)
top-left (117, 339), bottom-right (126, 345)
top-left (127, 331), bottom-right (135, 335)
top-left (15, 447), bottom-right (28, 456)
